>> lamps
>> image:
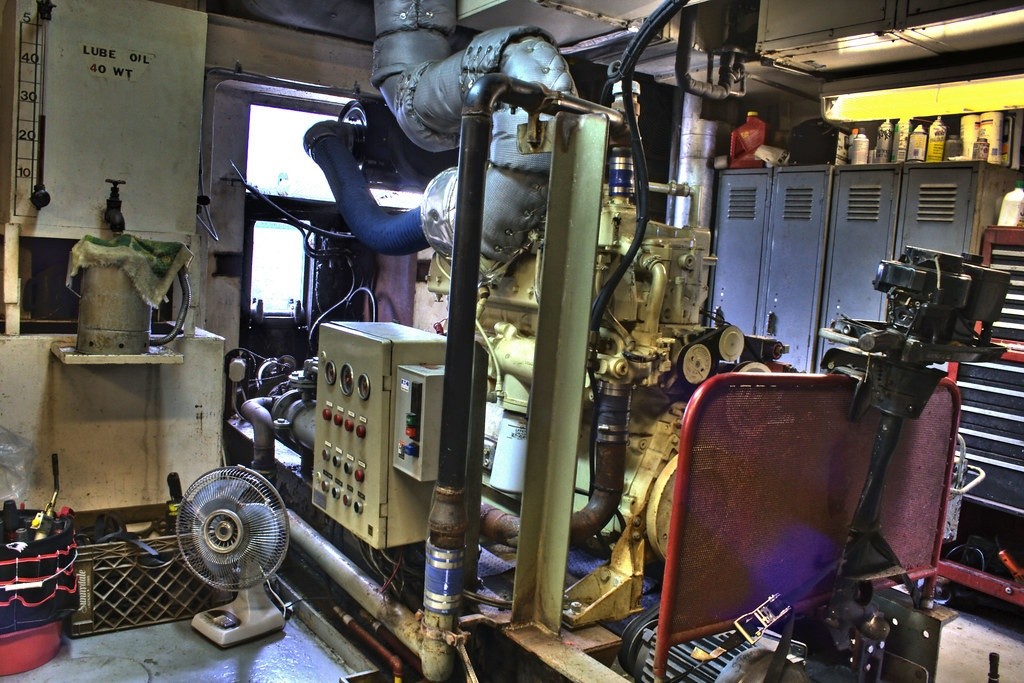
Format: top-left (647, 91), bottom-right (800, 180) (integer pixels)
top-left (819, 58), bottom-right (1024, 128)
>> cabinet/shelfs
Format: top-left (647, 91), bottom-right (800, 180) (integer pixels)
top-left (709, 163), bottom-right (835, 375)
top-left (754, 0), bottom-right (1024, 76)
top-left (816, 161), bottom-right (1020, 373)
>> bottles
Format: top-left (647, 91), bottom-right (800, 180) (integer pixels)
top-left (845, 112), bottom-right (1004, 164)
top-left (729, 111), bottom-right (766, 168)
top-left (997, 178), bottom-right (1024, 227)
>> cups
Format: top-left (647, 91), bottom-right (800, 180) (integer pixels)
top-left (756, 144), bottom-right (790, 166)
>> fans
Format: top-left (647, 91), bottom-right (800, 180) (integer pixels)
top-left (176, 464), bottom-right (292, 648)
